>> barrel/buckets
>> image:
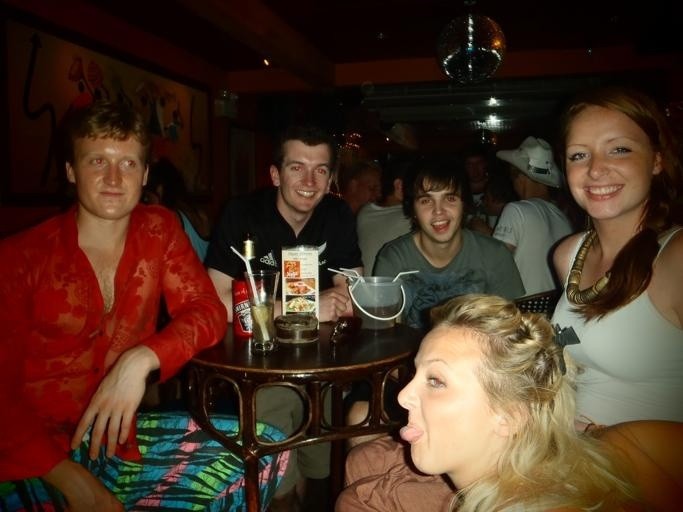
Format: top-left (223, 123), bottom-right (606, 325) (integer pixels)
top-left (347, 274), bottom-right (408, 331)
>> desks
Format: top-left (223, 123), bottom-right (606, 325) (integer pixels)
top-left (183, 322), bottom-right (420, 511)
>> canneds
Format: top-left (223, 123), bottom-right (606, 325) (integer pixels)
top-left (233, 279), bottom-right (262, 343)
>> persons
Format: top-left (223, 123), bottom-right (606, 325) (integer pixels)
top-left (203, 89), bottom-right (683, 334)
top-left (3, 97), bottom-right (228, 512)
top-left (395, 294), bottom-right (643, 512)
top-left (337, 93), bottom-right (682, 512)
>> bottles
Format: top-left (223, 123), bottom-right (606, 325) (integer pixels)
top-left (231, 230), bottom-right (263, 338)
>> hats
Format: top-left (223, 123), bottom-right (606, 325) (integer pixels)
top-left (496, 136), bottom-right (564, 188)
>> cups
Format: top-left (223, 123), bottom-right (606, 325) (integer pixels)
top-left (242, 269), bottom-right (279, 353)
top-left (343, 275), bottom-right (402, 330)
